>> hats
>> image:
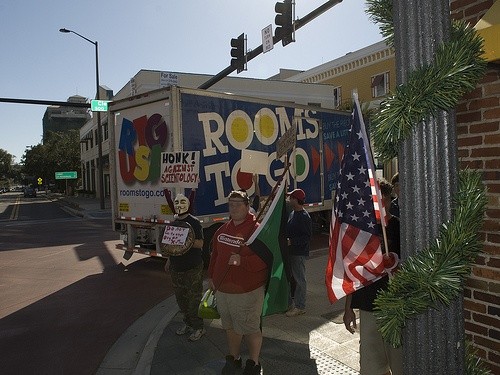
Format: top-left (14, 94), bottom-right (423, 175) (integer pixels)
top-left (287, 189), bottom-right (305, 201)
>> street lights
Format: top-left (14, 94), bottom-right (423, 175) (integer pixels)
top-left (59, 28), bottom-right (105, 209)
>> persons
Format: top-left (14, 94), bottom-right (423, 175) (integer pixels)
top-left (344, 179), bottom-right (405, 375)
top-left (161, 193), bottom-right (206, 341)
top-left (208, 191), bottom-right (271, 375)
top-left (285, 189), bottom-right (311, 318)
top-left (389, 172), bottom-right (400, 217)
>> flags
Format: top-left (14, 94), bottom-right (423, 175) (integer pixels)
top-left (197, 186), bottom-right (290, 320)
top-left (325, 100), bottom-right (388, 306)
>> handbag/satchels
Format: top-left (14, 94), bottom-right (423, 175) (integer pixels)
top-left (198, 288), bottom-right (220, 319)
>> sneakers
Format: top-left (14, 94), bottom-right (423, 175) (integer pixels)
top-left (285, 306), bottom-right (305, 317)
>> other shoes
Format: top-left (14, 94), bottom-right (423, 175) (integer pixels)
top-left (243, 359), bottom-right (263, 375)
top-left (188, 327), bottom-right (207, 341)
top-left (220, 355), bottom-right (242, 375)
top-left (176, 324), bottom-right (192, 335)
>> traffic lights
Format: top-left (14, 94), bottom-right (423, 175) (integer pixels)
top-left (275, 0), bottom-right (292, 47)
top-left (231, 33), bottom-right (244, 74)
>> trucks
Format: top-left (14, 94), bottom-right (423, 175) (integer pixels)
top-left (108, 85), bottom-right (387, 268)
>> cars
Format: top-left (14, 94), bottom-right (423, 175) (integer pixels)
top-left (0, 186), bottom-right (23, 194)
top-left (24, 188), bottom-right (36, 197)
